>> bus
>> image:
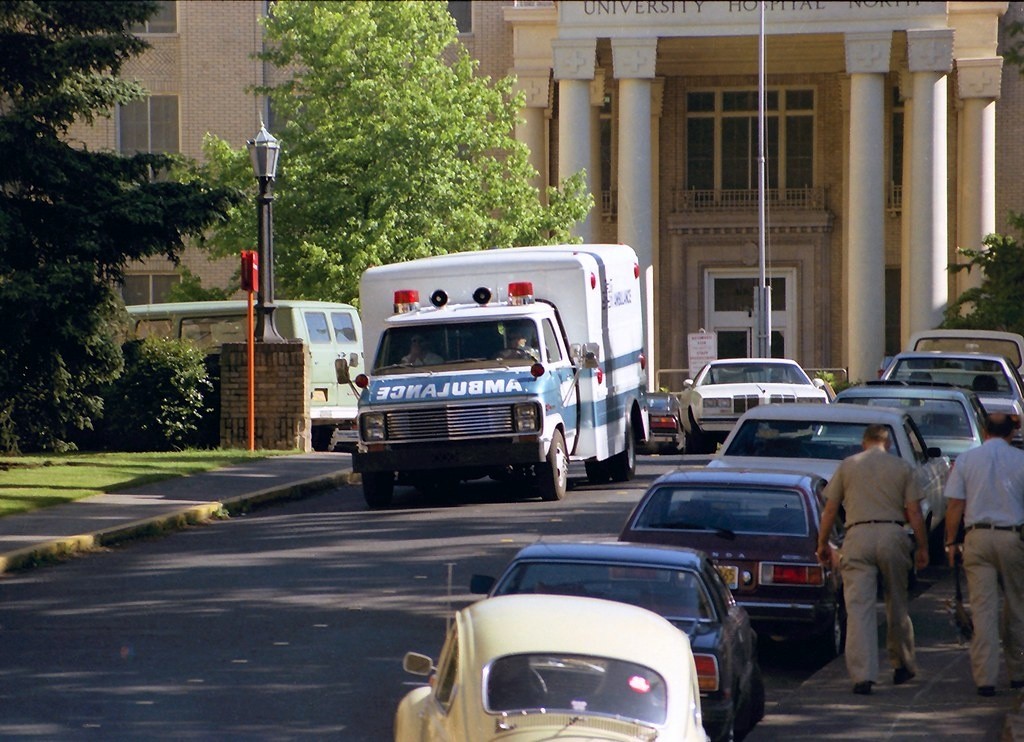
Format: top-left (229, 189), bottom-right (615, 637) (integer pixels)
top-left (124, 298), bottom-right (364, 452)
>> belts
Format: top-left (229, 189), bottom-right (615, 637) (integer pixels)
top-left (845, 520), bottom-right (904, 530)
top-left (964, 524), bottom-right (1022, 533)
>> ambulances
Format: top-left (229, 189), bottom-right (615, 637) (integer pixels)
top-left (334, 243), bottom-right (651, 511)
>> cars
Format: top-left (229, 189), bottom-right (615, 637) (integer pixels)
top-left (471, 541), bottom-right (767, 742)
top-left (811, 330), bottom-right (1023, 468)
top-left (607, 465), bottom-right (848, 668)
top-left (688, 402), bottom-right (952, 597)
top-left (394, 592), bottom-right (717, 742)
top-left (642, 389), bottom-right (680, 449)
top-left (678, 356), bottom-right (830, 455)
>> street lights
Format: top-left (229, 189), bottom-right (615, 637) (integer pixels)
top-left (243, 117), bottom-right (284, 342)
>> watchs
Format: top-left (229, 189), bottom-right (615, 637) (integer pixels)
top-left (945, 542), bottom-right (955, 551)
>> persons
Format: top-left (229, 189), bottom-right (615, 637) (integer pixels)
top-left (401, 332), bottom-right (443, 366)
top-left (494, 328), bottom-right (541, 363)
top-left (817, 424), bottom-right (932, 696)
top-left (942, 411), bottom-right (1024, 696)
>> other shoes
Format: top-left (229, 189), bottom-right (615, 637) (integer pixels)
top-left (977, 686), bottom-right (995, 697)
top-left (853, 680), bottom-right (876, 696)
top-left (893, 666), bottom-right (915, 685)
top-left (1009, 680), bottom-right (1024, 689)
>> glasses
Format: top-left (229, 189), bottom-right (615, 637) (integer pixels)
top-left (412, 338), bottom-right (425, 343)
top-left (508, 335), bottom-right (524, 341)
